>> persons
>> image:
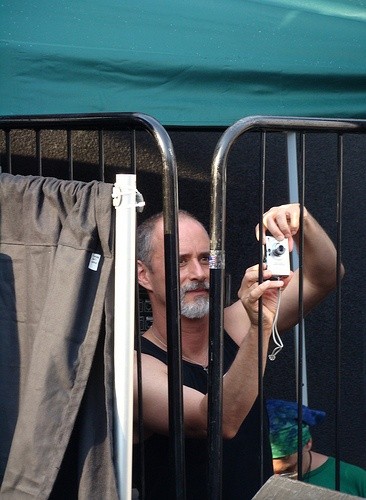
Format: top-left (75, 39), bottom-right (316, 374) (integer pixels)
top-left (132, 203), bottom-right (345, 500)
top-left (266, 399), bottom-right (366, 498)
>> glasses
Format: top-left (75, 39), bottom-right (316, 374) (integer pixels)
top-left (281, 472), bottom-right (299, 478)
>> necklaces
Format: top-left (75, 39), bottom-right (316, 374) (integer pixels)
top-left (308, 450), bottom-right (313, 482)
top-left (149, 325), bottom-right (205, 366)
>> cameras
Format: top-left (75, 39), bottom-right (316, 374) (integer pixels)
top-left (263, 236), bottom-right (290, 280)
